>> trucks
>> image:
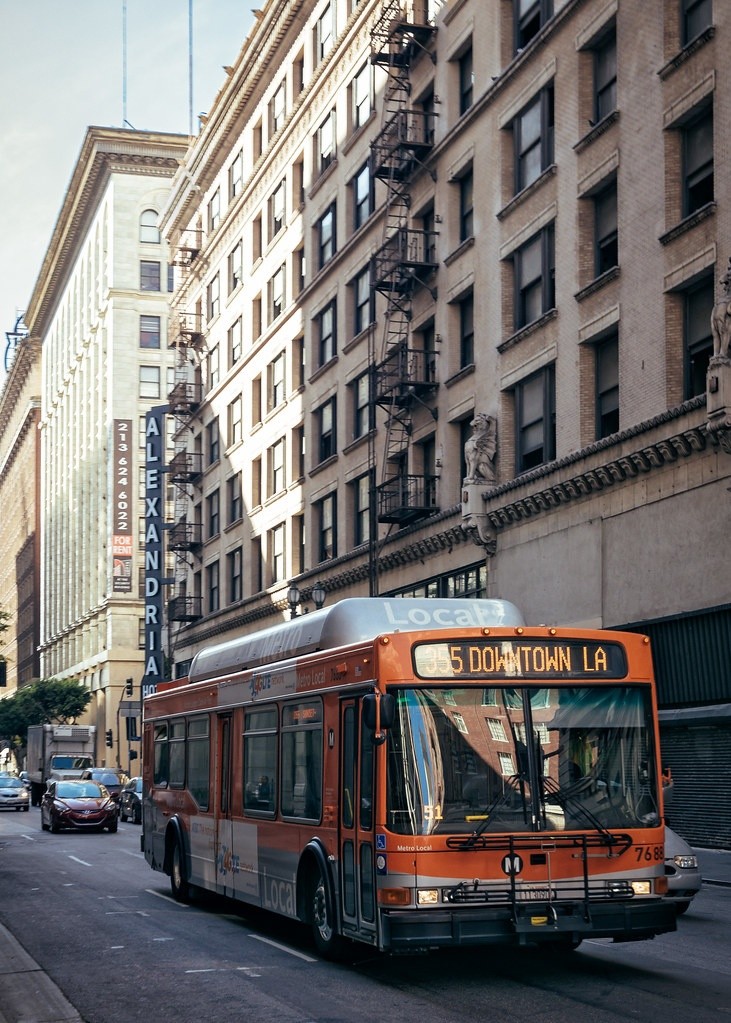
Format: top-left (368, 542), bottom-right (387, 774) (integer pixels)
top-left (25, 724), bottom-right (97, 807)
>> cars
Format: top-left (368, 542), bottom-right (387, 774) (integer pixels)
top-left (596, 780), bottom-right (702, 915)
top-left (0, 771), bottom-right (32, 812)
top-left (41, 781), bottom-right (119, 833)
top-left (118, 777), bottom-right (144, 825)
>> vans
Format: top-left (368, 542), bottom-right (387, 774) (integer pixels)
top-left (80, 767), bottom-right (126, 800)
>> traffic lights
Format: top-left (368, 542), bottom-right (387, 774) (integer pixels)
top-left (131, 749), bottom-right (139, 760)
top-left (105, 728), bottom-right (112, 748)
top-left (126, 677), bottom-right (133, 696)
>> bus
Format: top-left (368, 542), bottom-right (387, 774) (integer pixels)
top-left (139, 598), bottom-right (677, 972)
